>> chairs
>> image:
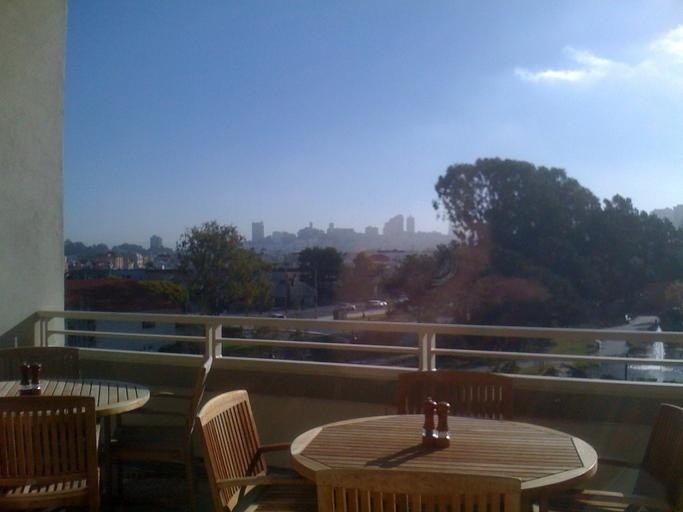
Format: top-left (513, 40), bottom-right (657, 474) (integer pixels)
top-left (316, 467), bottom-right (522, 512)
top-left (538, 403), bottom-right (683, 512)
top-left (197, 389), bottom-right (311, 511)
top-left (407, 371), bottom-right (515, 422)
top-left (103, 356), bottom-right (213, 511)
top-left (1, 348), bottom-right (82, 372)
top-left (1, 395), bottom-right (101, 512)
